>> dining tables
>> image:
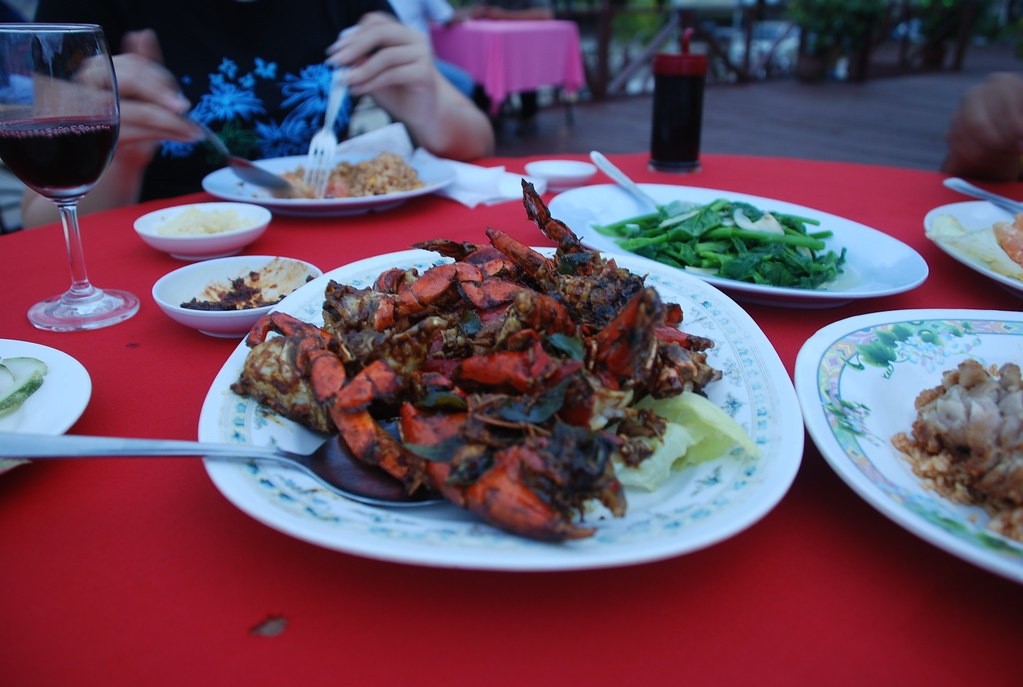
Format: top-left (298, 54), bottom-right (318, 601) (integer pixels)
top-left (431, 14), bottom-right (585, 153)
top-left (0, 154), bottom-right (1022, 687)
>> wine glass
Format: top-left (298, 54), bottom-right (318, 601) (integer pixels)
top-left (0, 22), bottom-right (140, 332)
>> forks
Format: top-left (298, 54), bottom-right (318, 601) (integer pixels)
top-left (304, 66), bottom-right (346, 199)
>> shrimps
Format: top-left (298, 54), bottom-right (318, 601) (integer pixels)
top-left (231, 176), bottom-right (728, 545)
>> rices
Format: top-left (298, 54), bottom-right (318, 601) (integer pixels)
top-left (277, 152), bottom-right (425, 199)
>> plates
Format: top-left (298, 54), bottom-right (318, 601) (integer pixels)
top-left (794, 308), bottom-right (1023, 586)
top-left (525, 160), bottom-right (597, 192)
top-left (133, 202), bottom-right (272, 262)
top-left (924, 198), bottom-right (1023, 291)
top-left (543, 182), bottom-right (929, 308)
top-left (151, 256), bottom-right (324, 339)
top-left (0, 338), bottom-right (92, 476)
top-left (200, 153), bottom-right (456, 216)
top-left (190, 244), bottom-right (806, 573)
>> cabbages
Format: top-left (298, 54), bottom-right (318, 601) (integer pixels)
top-left (601, 390), bottom-right (761, 490)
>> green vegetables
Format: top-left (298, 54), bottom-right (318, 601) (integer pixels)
top-left (591, 197), bottom-right (849, 291)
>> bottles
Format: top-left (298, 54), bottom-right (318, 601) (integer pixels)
top-left (650, 28), bottom-right (708, 175)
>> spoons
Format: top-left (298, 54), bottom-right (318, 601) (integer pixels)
top-left (181, 111), bottom-right (292, 189)
top-left (588, 148), bottom-right (689, 219)
top-left (0, 430), bottom-right (453, 506)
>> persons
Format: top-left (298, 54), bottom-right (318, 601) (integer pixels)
top-left (472, 0), bottom-right (554, 136)
top-left (940, 70), bottom-right (1023, 181)
top-left (22, 0), bottom-right (493, 229)
top-left (389, 0), bottom-right (489, 110)
